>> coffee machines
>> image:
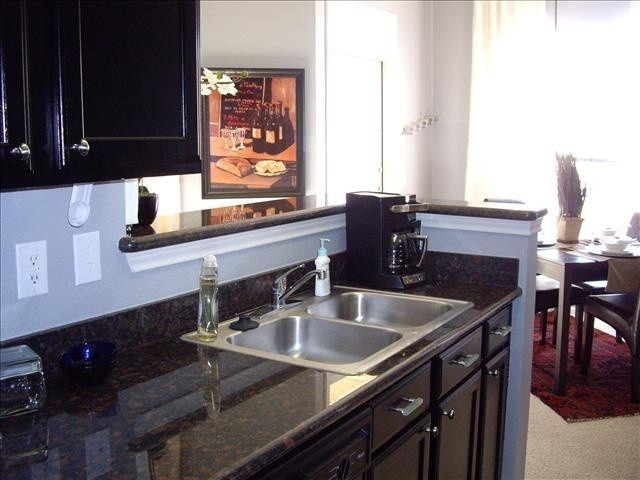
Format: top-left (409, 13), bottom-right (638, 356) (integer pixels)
top-left (345, 191), bottom-right (431, 291)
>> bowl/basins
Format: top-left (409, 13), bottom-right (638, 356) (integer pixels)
top-left (604, 243), bottom-right (629, 252)
top-left (59, 340), bottom-right (117, 385)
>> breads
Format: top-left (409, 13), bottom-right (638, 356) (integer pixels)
top-left (215, 158), bottom-right (252, 177)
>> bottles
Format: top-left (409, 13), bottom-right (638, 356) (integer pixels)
top-left (251, 101), bottom-right (292, 155)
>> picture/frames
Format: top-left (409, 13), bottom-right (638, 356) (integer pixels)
top-left (201, 67), bottom-right (307, 199)
top-left (201, 196), bottom-right (303, 227)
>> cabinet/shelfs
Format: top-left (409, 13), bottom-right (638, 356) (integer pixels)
top-left (1, 0), bottom-right (203, 194)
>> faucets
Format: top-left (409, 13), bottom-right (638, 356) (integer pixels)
top-left (267, 261), bottom-right (325, 309)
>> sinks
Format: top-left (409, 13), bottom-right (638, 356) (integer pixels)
top-left (280, 284), bottom-right (476, 337)
top-left (179, 312), bottom-right (417, 378)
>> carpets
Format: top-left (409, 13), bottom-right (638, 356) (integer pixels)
top-left (531, 311), bottom-right (640, 423)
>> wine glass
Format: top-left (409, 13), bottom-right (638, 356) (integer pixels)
top-left (221, 127), bottom-right (246, 152)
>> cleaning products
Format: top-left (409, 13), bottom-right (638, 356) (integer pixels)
top-left (313, 237), bottom-right (334, 296)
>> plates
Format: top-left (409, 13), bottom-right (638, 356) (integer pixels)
top-left (253, 171), bottom-right (288, 177)
top-left (538, 242), bottom-right (556, 247)
top-left (601, 248), bottom-right (630, 253)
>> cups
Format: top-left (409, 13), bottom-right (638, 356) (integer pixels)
top-left (0, 411), bottom-right (50, 472)
top-left (0, 344), bottom-right (46, 418)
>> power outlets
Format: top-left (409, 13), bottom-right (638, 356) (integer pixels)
top-left (15, 240), bottom-right (49, 300)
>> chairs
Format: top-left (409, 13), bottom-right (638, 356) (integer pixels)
top-left (536, 275), bottom-right (584, 365)
top-left (579, 256), bottom-right (640, 405)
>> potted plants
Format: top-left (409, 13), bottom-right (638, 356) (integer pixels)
top-left (556, 154), bottom-right (587, 243)
